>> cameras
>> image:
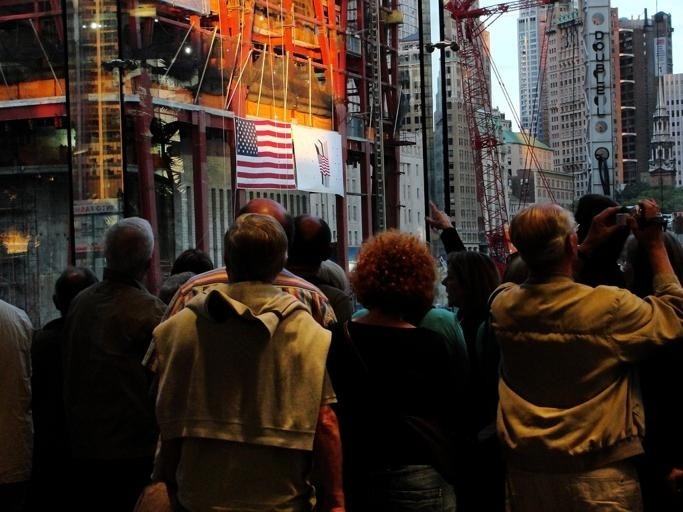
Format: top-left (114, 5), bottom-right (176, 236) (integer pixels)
top-left (614, 204), bottom-right (667, 232)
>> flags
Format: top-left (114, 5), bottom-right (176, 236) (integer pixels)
top-left (234, 116), bottom-right (296, 190)
top-left (292, 124), bottom-right (344, 197)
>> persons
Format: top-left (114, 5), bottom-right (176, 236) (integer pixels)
top-left (330, 231), bottom-right (480, 512)
top-left (425, 199), bottom-right (534, 286)
top-left (487, 199), bottom-right (683, 512)
top-left (0, 298), bottom-right (33, 512)
top-left (153, 213), bottom-right (345, 512)
top-left (31, 268), bottom-right (100, 359)
top-left (287, 215), bottom-right (354, 322)
top-left (160, 199), bottom-right (336, 347)
top-left (440, 250), bottom-right (505, 386)
top-left (158, 247), bottom-right (216, 303)
top-left (666, 215), bottom-right (683, 249)
top-left (350, 306), bottom-right (469, 357)
top-left (618, 230), bottom-right (683, 297)
top-left (33, 217), bottom-right (169, 512)
top-left (572, 194), bottom-right (631, 287)
